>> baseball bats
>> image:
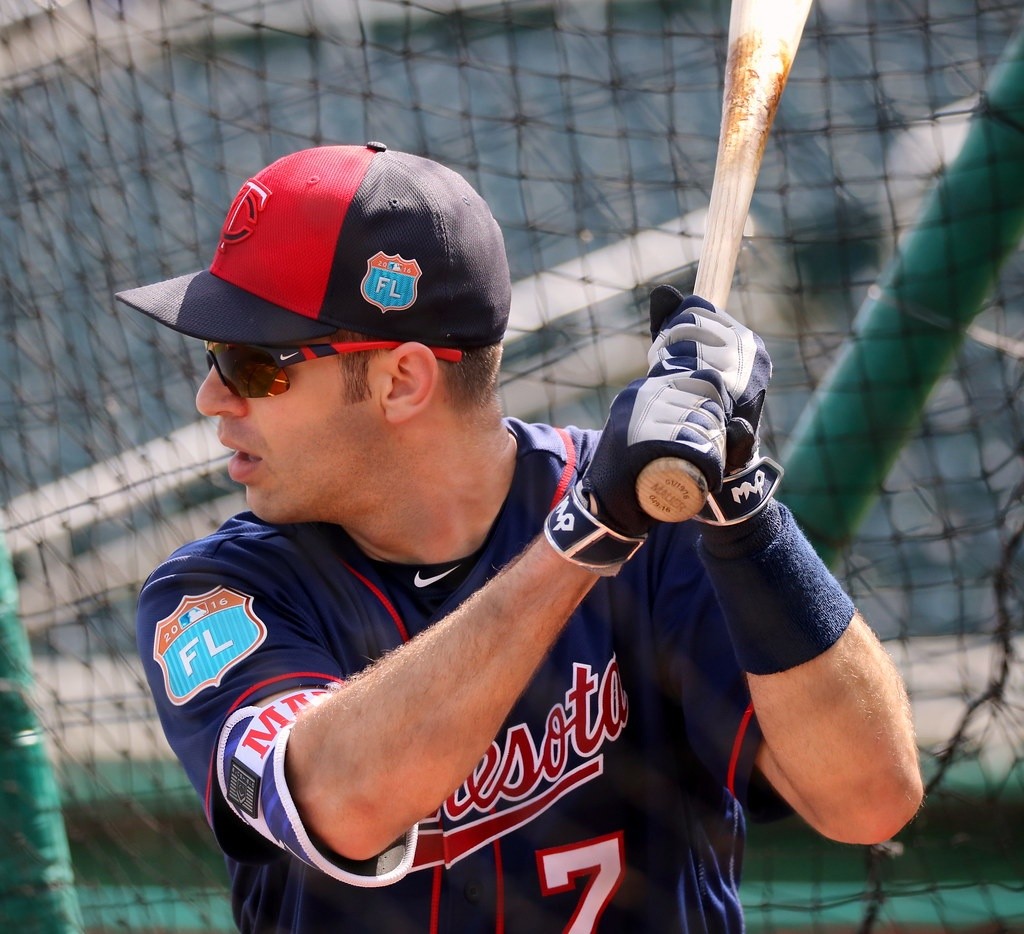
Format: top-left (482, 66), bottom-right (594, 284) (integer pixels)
top-left (632, 0), bottom-right (818, 522)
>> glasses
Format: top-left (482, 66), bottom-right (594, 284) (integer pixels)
top-left (203, 337), bottom-right (462, 399)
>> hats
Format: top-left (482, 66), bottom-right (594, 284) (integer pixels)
top-left (113, 141), bottom-right (512, 351)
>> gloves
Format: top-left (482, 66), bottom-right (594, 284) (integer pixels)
top-left (542, 369), bottom-right (756, 577)
top-left (646, 284), bottom-right (785, 527)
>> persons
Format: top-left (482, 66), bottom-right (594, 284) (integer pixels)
top-left (113, 142), bottom-right (924, 934)
